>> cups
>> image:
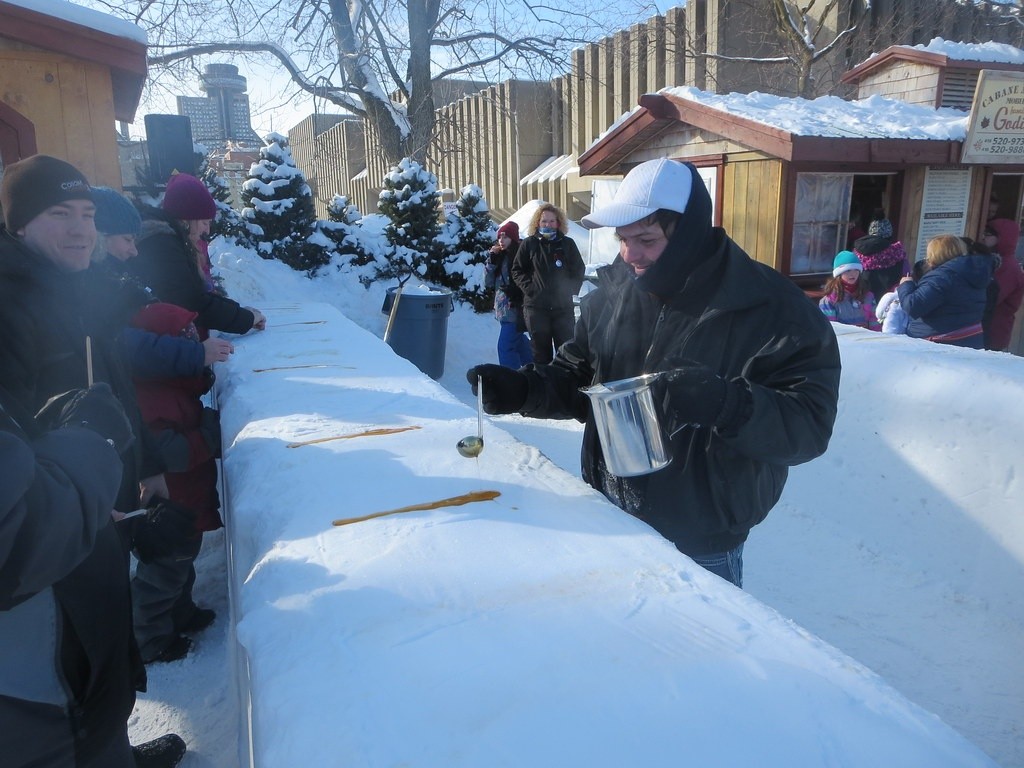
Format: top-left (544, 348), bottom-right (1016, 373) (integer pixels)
top-left (902, 278), bottom-right (912, 281)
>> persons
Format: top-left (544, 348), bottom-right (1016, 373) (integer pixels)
top-left (467, 154), bottom-right (842, 589)
top-left (483, 221), bottom-right (533, 370)
top-left (820, 199), bottom-right (1024, 355)
top-left (0, 153), bottom-right (268, 768)
top-left (511, 203), bottom-right (586, 368)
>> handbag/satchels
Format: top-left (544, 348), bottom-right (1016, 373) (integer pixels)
top-left (516, 301), bottom-right (528, 332)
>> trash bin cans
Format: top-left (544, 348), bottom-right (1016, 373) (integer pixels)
top-left (381, 287), bottom-right (455, 379)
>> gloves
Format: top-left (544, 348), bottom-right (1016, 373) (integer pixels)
top-left (653, 356), bottom-right (726, 429)
top-left (35, 382), bottom-right (135, 457)
top-left (466, 364), bottom-right (529, 414)
top-left (200, 406), bottom-right (221, 458)
top-left (132, 493), bottom-right (197, 567)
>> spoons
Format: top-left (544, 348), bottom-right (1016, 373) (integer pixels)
top-left (456, 374), bottom-right (485, 459)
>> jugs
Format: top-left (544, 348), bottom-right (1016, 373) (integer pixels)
top-left (578, 373), bottom-right (692, 477)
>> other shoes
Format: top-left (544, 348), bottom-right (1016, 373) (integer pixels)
top-left (144, 637), bottom-right (188, 665)
top-left (131, 733), bottom-right (186, 768)
top-left (172, 601), bottom-right (215, 634)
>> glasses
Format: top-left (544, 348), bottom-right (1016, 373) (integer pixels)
top-left (982, 233), bottom-right (994, 240)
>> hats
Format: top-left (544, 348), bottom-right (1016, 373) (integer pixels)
top-left (90, 186), bottom-right (143, 235)
top-left (833, 250), bottom-right (863, 278)
top-left (497, 221), bottom-right (519, 242)
top-left (581, 157), bottom-right (692, 229)
top-left (0, 153), bottom-right (96, 234)
top-left (162, 173), bottom-right (217, 220)
top-left (868, 209), bottom-right (892, 238)
